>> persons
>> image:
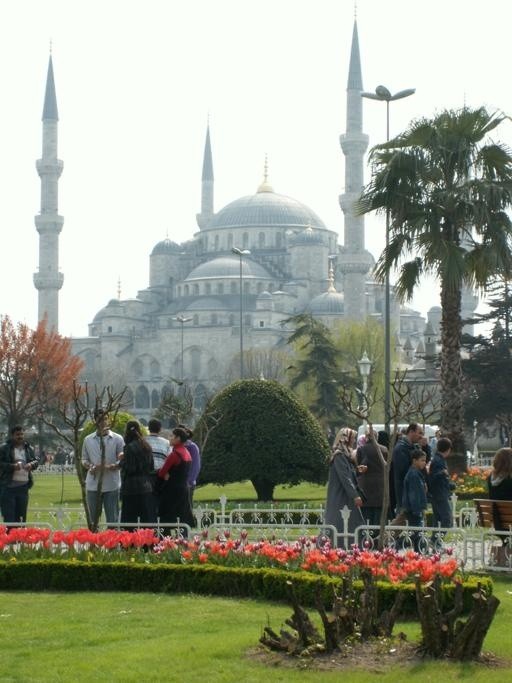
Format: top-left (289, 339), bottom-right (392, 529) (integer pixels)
top-left (466, 448), bottom-right (472, 468)
top-left (487, 448), bottom-right (510, 545)
top-left (0, 409), bottom-right (201, 537)
top-left (324, 424), bottom-right (455, 549)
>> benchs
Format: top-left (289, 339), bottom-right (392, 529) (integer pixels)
top-left (474, 498), bottom-right (511, 573)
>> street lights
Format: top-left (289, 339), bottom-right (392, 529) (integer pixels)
top-left (230, 245), bottom-right (253, 380)
top-left (354, 80), bottom-right (420, 435)
top-left (356, 347), bottom-right (374, 438)
top-left (169, 315), bottom-right (195, 377)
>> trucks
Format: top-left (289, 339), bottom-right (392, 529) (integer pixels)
top-left (353, 422), bottom-right (476, 463)
